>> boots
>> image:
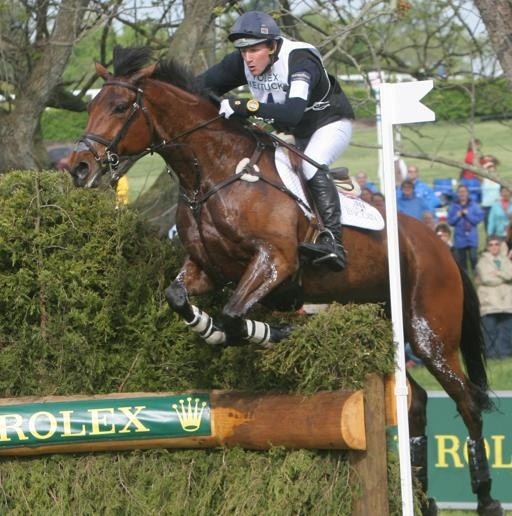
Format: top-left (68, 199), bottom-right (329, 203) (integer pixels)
top-left (298, 162), bottom-right (349, 273)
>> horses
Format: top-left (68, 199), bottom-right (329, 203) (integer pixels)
top-left (68, 44), bottom-right (504, 516)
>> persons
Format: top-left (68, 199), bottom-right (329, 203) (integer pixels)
top-left (358, 138), bottom-right (511, 364)
top-left (189, 9), bottom-right (357, 271)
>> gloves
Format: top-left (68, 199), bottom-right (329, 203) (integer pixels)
top-left (217, 95), bottom-right (254, 123)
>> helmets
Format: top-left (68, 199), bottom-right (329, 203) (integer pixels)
top-left (227, 10), bottom-right (283, 48)
